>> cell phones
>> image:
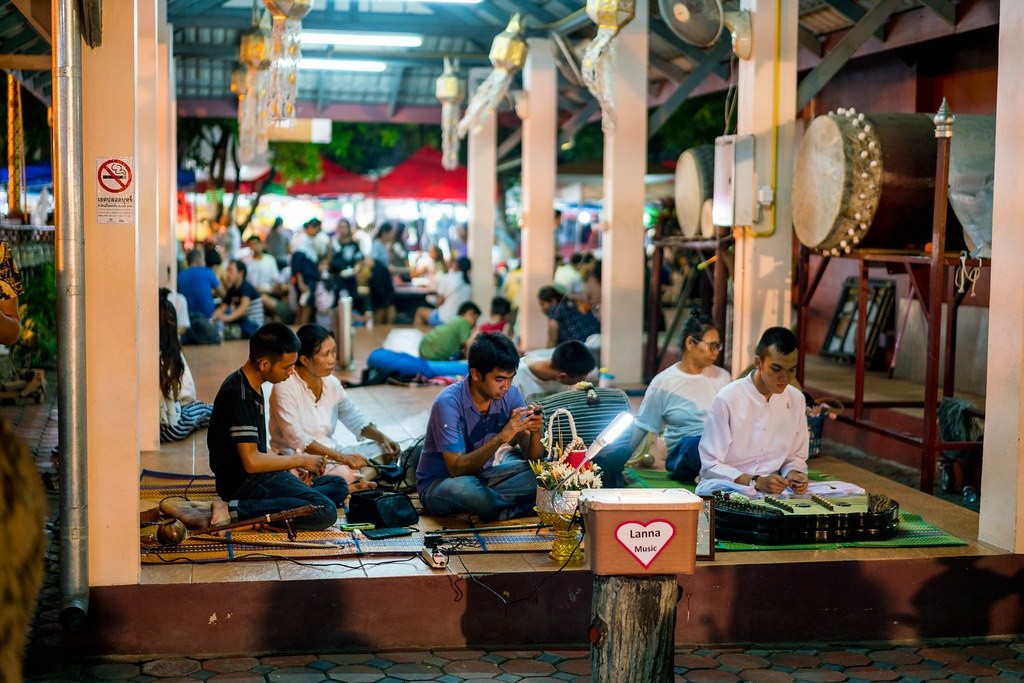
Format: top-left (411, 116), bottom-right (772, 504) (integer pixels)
top-left (526, 409), bottom-right (542, 421)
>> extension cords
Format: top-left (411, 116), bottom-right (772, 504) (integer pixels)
top-left (421, 547), bottom-right (447, 568)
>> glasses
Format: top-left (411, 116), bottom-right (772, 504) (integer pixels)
top-left (692, 336), bottom-right (724, 353)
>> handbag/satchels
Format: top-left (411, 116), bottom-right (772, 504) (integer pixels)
top-left (373, 493), bottom-right (418, 530)
top-left (311, 270), bottom-right (336, 312)
top-left (346, 489), bottom-right (383, 527)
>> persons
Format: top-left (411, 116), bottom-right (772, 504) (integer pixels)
top-left (415, 330), bottom-right (544, 520)
top-left (206, 321), bottom-right (351, 531)
top-left (624, 310), bottom-right (733, 485)
top-left (270, 325), bottom-right (401, 492)
top-left (695, 327), bottom-right (866, 499)
top-left (158, 207), bottom-right (602, 443)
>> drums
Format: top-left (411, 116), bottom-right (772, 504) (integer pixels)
top-left (527, 386), bottom-right (635, 457)
top-left (626, 431), bottom-right (652, 466)
top-left (790, 106), bottom-right (963, 257)
top-left (674, 143), bottom-right (730, 240)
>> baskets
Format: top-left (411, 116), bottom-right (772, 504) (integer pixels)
top-left (534, 409), bottom-right (598, 514)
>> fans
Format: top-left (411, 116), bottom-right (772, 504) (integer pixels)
top-left (657, 0), bottom-right (751, 58)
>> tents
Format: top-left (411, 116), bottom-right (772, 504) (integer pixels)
top-left (1, 141), bottom-right (500, 237)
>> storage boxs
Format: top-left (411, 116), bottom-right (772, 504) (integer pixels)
top-left (578, 487), bottom-right (704, 575)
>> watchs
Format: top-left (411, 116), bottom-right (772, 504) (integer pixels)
top-left (748, 474), bottom-right (761, 493)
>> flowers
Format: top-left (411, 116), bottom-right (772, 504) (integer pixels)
top-left (527, 445), bottom-right (606, 490)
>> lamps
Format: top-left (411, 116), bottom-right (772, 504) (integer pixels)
top-left (551, 411), bottom-right (636, 523)
